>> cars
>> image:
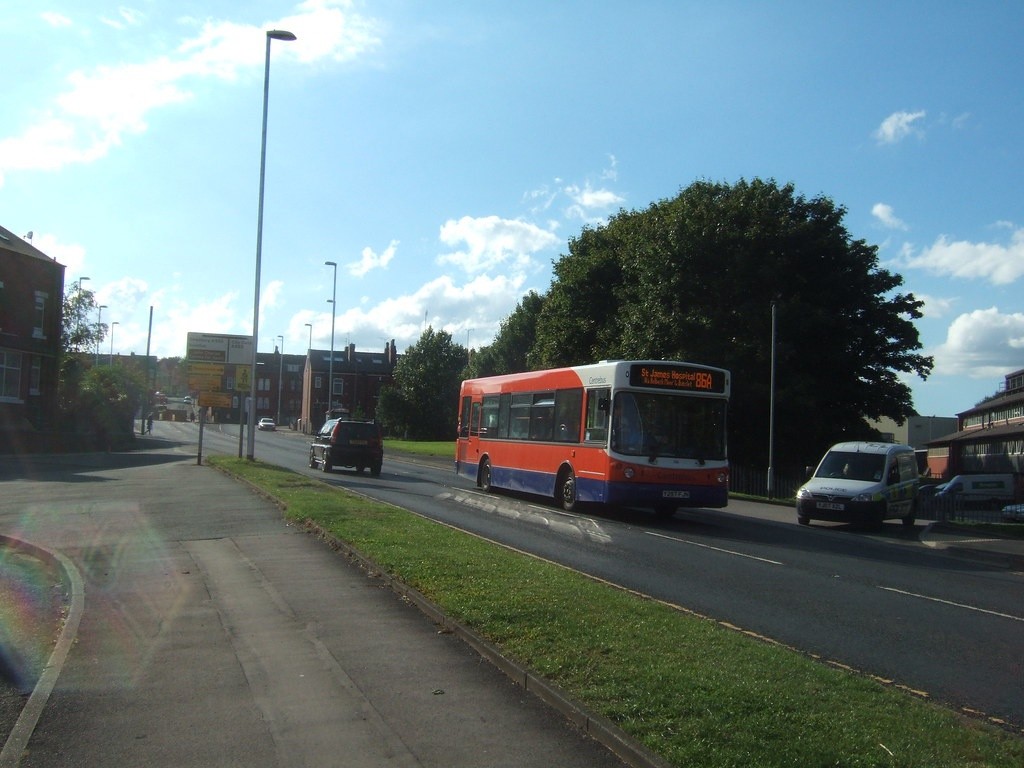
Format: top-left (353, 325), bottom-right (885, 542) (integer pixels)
top-left (919, 482), bottom-right (950, 493)
top-left (257, 417), bottom-right (276, 431)
top-left (1002, 505), bottom-right (1024, 514)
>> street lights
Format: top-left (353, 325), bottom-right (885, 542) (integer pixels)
top-left (305, 324), bottom-right (313, 434)
top-left (325, 261), bottom-right (337, 416)
top-left (110, 321), bottom-right (119, 367)
top-left (245, 29), bottom-right (298, 460)
top-left (96, 305), bottom-right (109, 367)
top-left (277, 335), bottom-right (284, 426)
top-left (75, 276), bottom-right (91, 352)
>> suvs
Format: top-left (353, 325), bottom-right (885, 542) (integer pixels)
top-left (308, 417), bottom-right (383, 477)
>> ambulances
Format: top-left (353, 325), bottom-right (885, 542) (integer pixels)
top-left (795, 441), bottom-right (920, 532)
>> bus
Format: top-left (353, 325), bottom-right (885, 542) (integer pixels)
top-left (453, 359), bottom-right (731, 513)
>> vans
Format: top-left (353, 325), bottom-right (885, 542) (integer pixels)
top-left (936, 474), bottom-right (1013, 501)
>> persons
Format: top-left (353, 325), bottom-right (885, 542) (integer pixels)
top-left (190, 412), bottom-right (195, 422)
top-left (146, 414), bottom-right (153, 434)
